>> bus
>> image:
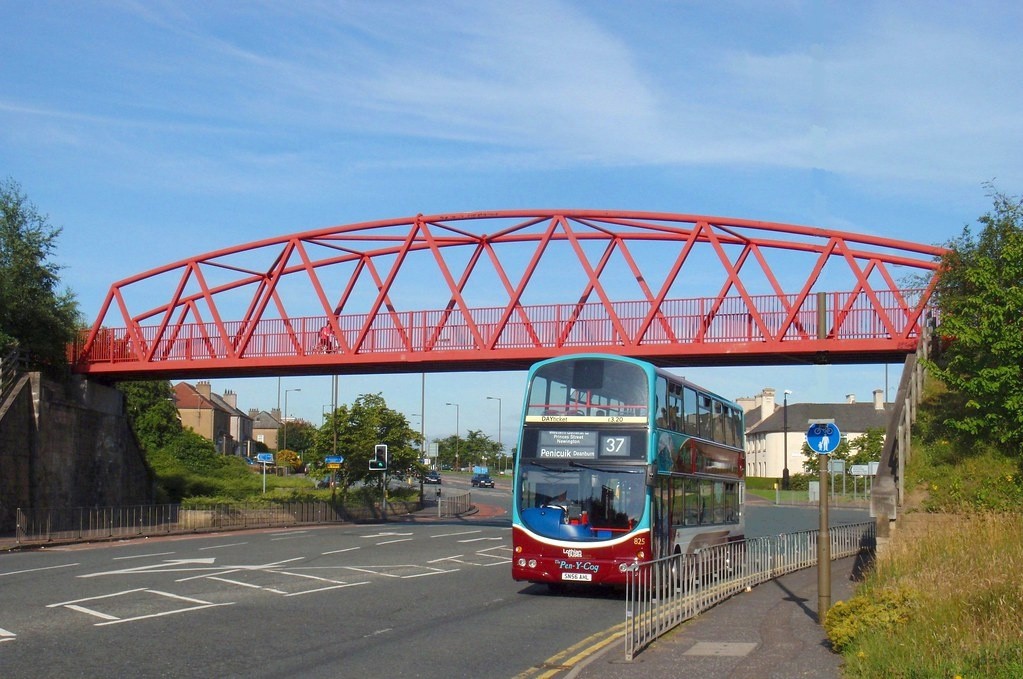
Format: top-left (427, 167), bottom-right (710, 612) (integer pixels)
top-left (509, 353), bottom-right (748, 591)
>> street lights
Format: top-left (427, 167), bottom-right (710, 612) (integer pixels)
top-left (284, 388), bottom-right (301, 448)
top-left (446, 403), bottom-right (459, 471)
top-left (486, 396), bottom-right (501, 475)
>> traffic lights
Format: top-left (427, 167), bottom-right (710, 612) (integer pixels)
top-left (369, 445), bottom-right (388, 470)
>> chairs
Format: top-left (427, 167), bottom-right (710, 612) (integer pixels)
top-left (670, 506), bottom-right (737, 526)
top-left (538, 409), bottom-right (635, 416)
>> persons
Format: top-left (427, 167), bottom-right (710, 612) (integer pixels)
top-left (690, 496), bottom-right (708, 523)
top-left (317, 320), bottom-right (339, 354)
top-left (548, 487), bottom-right (576, 506)
top-left (601, 509), bottom-right (620, 527)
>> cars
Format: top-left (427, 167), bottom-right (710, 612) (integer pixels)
top-left (469, 473), bottom-right (495, 488)
top-left (420, 469), bottom-right (442, 484)
